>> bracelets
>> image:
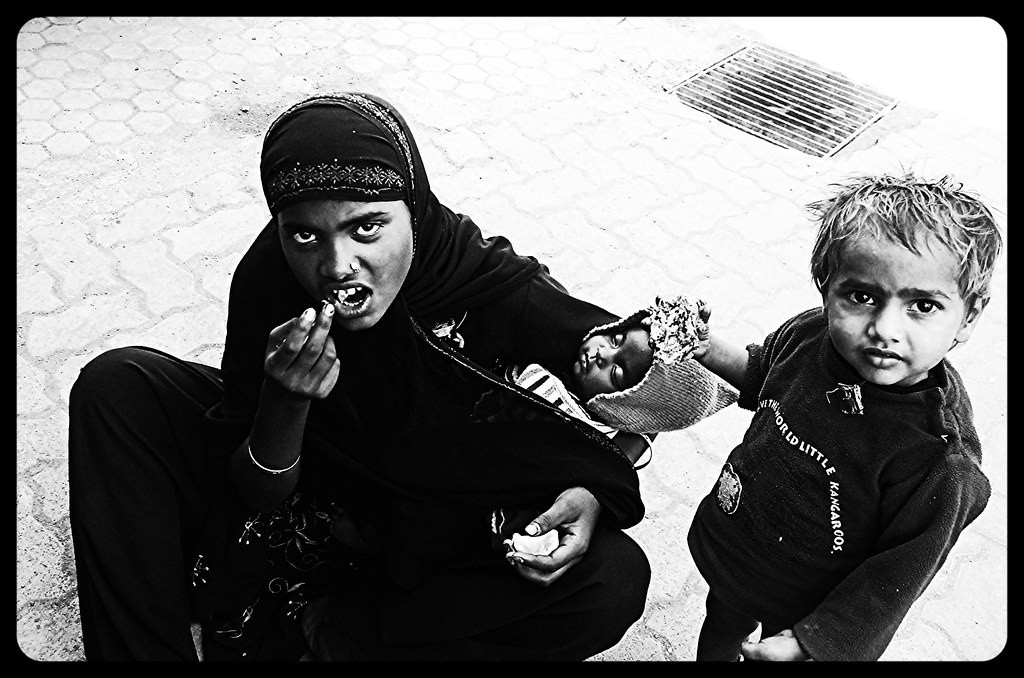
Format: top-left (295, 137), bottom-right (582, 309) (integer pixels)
top-left (246, 434), bottom-right (300, 473)
top-left (611, 429), bottom-right (653, 470)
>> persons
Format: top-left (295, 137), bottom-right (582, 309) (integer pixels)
top-left (66, 89), bottom-right (656, 663)
top-left (189, 292), bottom-right (741, 661)
top-left (683, 170), bottom-right (1003, 661)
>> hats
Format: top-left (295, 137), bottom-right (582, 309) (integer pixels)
top-left (259, 105), bottom-right (409, 217)
